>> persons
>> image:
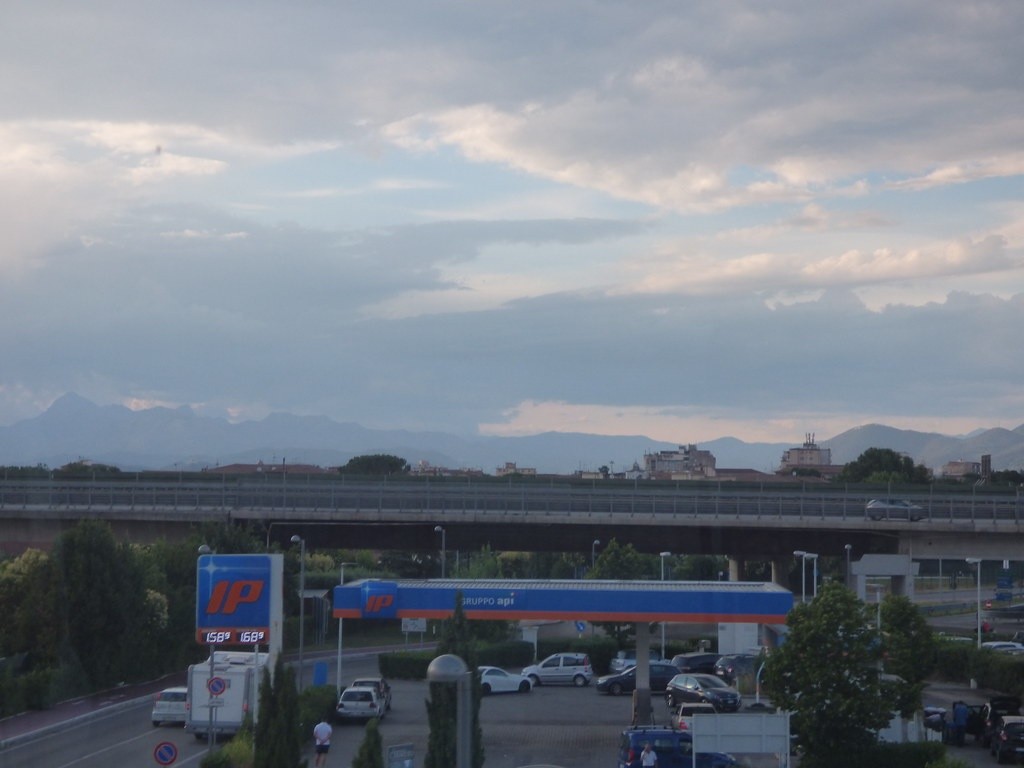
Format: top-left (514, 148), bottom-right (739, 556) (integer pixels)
top-left (640, 743), bottom-right (657, 768)
top-left (982, 618), bottom-right (990, 633)
top-left (953, 700), bottom-right (971, 747)
top-left (943, 707), bottom-right (955, 745)
top-left (314, 713), bottom-right (332, 768)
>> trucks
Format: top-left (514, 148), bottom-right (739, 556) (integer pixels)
top-left (186, 651), bottom-right (270, 740)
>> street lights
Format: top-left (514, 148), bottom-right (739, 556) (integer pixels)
top-left (335, 561), bottom-right (360, 705)
top-left (659, 551), bottom-right (674, 658)
top-left (591, 539), bottom-right (602, 570)
top-left (846, 544), bottom-right (854, 593)
top-left (434, 525), bottom-right (448, 578)
top-left (964, 556), bottom-right (982, 649)
top-left (290, 534), bottom-right (306, 674)
top-left (794, 549), bottom-right (807, 606)
top-left (803, 553), bottom-right (819, 599)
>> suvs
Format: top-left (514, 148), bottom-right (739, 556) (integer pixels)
top-left (620, 726), bottom-right (737, 768)
top-left (712, 653), bottom-right (771, 685)
top-left (609, 649), bottom-right (673, 674)
top-left (522, 652), bottom-right (594, 688)
top-left (672, 651), bottom-right (725, 676)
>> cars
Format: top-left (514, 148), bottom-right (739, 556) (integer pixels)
top-left (865, 498), bottom-right (929, 522)
top-left (981, 641), bottom-right (1024, 654)
top-left (671, 702), bottom-right (719, 732)
top-left (595, 664), bottom-right (684, 697)
top-left (337, 686), bottom-right (384, 723)
top-left (952, 699), bottom-right (1024, 764)
top-left (478, 666), bottom-right (534, 695)
top-left (352, 677), bottom-right (393, 713)
top-left (151, 688), bottom-right (188, 727)
top-left (664, 673), bottom-right (742, 714)
